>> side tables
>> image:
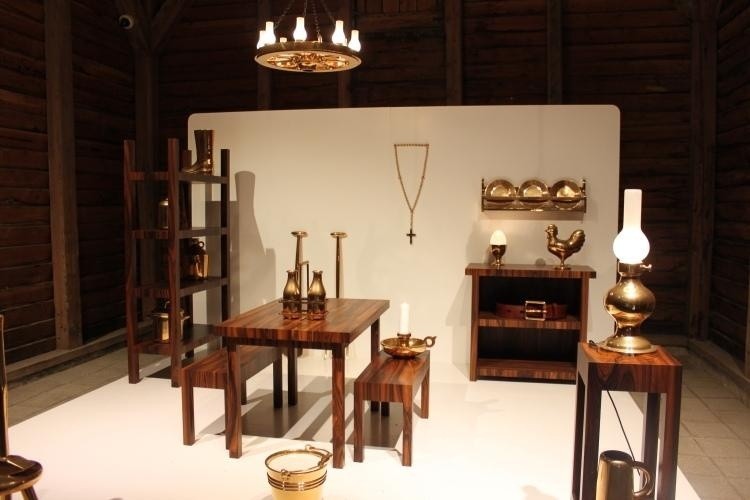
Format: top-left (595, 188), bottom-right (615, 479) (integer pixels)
top-left (572, 342), bottom-right (683, 500)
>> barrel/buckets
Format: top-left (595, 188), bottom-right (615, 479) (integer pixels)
top-left (265, 446), bottom-right (332, 500)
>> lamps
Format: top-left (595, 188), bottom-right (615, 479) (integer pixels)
top-left (489, 230), bottom-right (508, 272)
top-left (253, 0), bottom-right (363, 72)
top-left (600, 188), bottom-right (658, 355)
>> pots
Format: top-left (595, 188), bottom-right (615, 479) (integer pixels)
top-left (147, 301), bottom-right (191, 343)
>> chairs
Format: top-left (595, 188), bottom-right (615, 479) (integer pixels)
top-left (0, 313), bottom-right (43, 500)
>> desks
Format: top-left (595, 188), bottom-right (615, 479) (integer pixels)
top-left (212, 296), bottom-right (390, 470)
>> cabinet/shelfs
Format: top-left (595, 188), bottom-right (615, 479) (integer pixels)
top-left (464, 262), bottom-right (597, 383)
top-left (122, 138), bottom-right (231, 388)
top-left (481, 179), bottom-right (587, 214)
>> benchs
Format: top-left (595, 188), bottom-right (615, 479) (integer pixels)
top-left (180, 344), bottom-right (286, 450)
top-left (354, 349), bottom-right (430, 466)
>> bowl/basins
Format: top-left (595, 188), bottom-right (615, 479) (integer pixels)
top-left (552, 180), bottom-right (582, 214)
top-left (486, 180), bottom-right (515, 209)
top-left (517, 180), bottom-right (548, 212)
